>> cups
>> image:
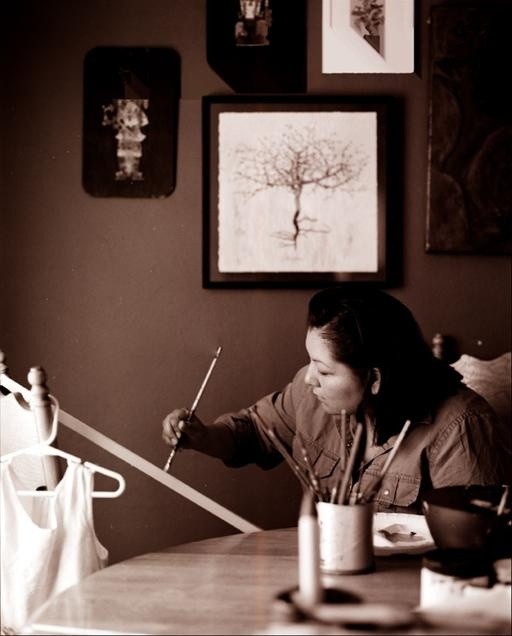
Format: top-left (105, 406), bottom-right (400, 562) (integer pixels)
top-left (315, 498), bottom-right (375, 575)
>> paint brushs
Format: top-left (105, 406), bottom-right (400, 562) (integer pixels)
top-left (163, 345), bottom-right (224, 473)
top-left (338, 422), bottom-right (363, 506)
top-left (296, 429), bottom-right (326, 503)
top-left (266, 429), bottom-right (311, 493)
top-left (359, 419), bottom-right (412, 503)
top-left (295, 463), bottom-right (316, 495)
top-left (339, 407), bottom-right (347, 487)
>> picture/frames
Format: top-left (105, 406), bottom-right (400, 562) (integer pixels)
top-left (321, 0), bottom-right (415, 76)
top-left (80, 44), bottom-right (182, 200)
top-left (200, 92), bottom-right (407, 288)
top-left (424, 1), bottom-right (511, 260)
top-left (205, 1), bottom-right (307, 96)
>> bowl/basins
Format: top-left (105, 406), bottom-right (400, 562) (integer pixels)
top-left (422, 484), bottom-right (512, 547)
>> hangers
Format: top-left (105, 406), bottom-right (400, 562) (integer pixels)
top-left (0, 394), bottom-right (126, 499)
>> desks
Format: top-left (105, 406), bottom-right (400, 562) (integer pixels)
top-left (25, 523), bottom-right (512, 635)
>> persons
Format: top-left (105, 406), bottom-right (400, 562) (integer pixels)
top-left (160, 279), bottom-right (510, 510)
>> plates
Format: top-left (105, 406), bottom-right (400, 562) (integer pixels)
top-left (371, 511), bottom-right (433, 556)
